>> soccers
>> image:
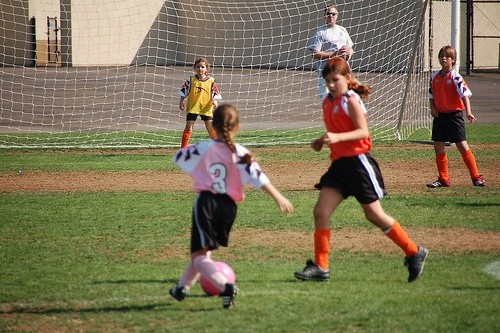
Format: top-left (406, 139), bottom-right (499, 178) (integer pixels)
top-left (200, 262), bottom-right (236, 295)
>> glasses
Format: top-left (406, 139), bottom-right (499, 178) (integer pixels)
top-left (326, 12), bottom-right (338, 17)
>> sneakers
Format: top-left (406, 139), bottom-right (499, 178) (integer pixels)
top-left (218, 283), bottom-right (239, 310)
top-left (472, 176), bottom-right (485, 186)
top-left (294, 259), bottom-right (330, 281)
top-left (169, 286), bottom-right (186, 301)
top-left (404, 244), bottom-right (430, 283)
top-left (426, 176), bottom-right (451, 187)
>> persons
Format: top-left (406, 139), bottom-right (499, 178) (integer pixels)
top-left (294, 57), bottom-right (429, 284)
top-left (426, 45), bottom-right (486, 188)
top-left (169, 102), bottom-right (295, 310)
top-left (312, 5), bottom-right (356, 121)
top-left (179, 56), bottom-right (222, 151)
